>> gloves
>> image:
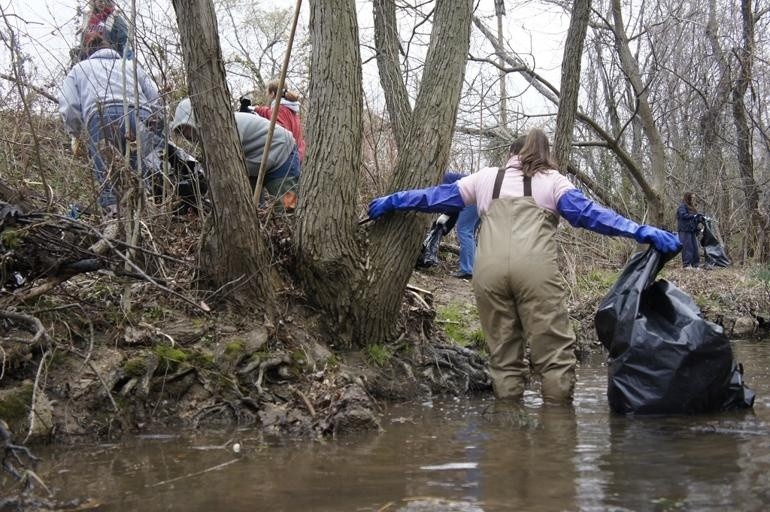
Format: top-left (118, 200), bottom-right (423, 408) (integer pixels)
top-left (557, 187), bottom-right (682, 253)
top-left (366, 182), bottom-right (465, 220)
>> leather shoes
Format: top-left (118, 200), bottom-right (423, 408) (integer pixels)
top-left (453, 272), bottom-right (472, 279)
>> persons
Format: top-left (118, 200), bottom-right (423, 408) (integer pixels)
top-left (365, 130), bottom-right (681, 406)
top-left (243, 79), bottom-right (305, 161)
top-left (442, 172), bottom-right (482, 279)
top-left (83, 0), bottom-right (133, 61)
top-left (62, 37), bottom-right (165, 218)
top-left (172, 96), bottom-right (302, 228)
top-left (676, 192), bottom-right (707, 272)
